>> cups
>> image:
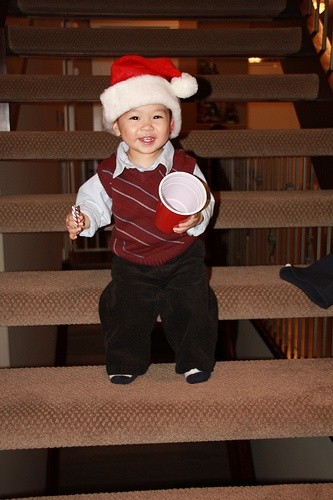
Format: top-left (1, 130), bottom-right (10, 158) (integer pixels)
top-left (156, 170), bottom-right (207, 235)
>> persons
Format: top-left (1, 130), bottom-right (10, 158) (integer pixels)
top-left (64, 55), bottom-right (218, 384)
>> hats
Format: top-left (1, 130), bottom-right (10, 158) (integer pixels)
top-left (99, 55), bottom-right (197, 139)
